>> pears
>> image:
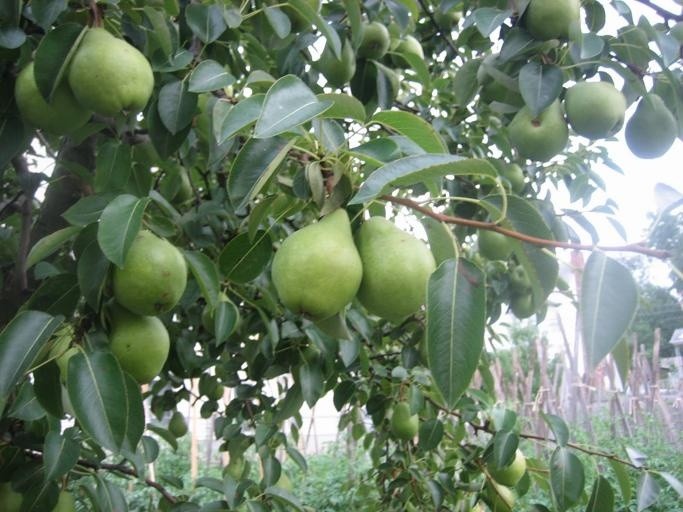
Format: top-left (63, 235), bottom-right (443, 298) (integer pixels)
top-left (1, 0), bottom-right (678, 512)
